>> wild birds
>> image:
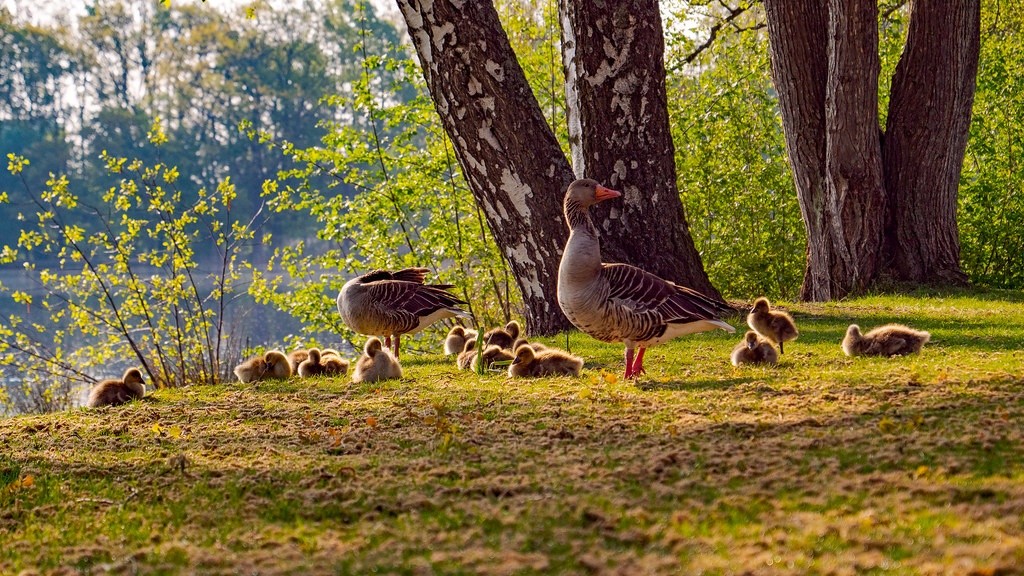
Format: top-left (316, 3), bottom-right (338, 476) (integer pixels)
top-left (730, 330), bottom-right (777, 371)
top-left (842, 322), bottom-right (932, 358)
top-left (443, 320), bottom-right (584, 378)
top-left (556, 179), bottom-right (738, 382)
top-left (745, 296), bottom-right (800, 355)
top-left (335, 267), bottom-right (474, 360)
top-left (352, 336), bottom-right (404, 385)
top-left (86, 368), bottom-right (147, 409)
top-left (233, 346), bottom-right (351, 385)
